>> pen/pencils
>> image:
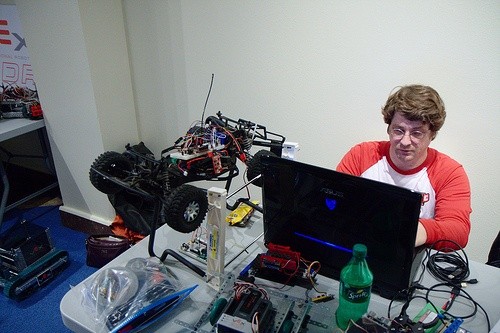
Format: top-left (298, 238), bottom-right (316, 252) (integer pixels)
top-left (441, 292), bottom-right (456, 310)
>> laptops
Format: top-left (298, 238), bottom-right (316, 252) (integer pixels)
top-left (259, 151), bottom-right (427, 303)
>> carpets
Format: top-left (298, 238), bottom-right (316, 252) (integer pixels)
top-left (0, 206), bottom-right (98, 333)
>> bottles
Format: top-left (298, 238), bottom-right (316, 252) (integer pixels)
top-left (335, 244), bottom-right (373, 330)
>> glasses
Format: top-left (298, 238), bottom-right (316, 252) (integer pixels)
top-left (387, 125), bottom-right (431, 139)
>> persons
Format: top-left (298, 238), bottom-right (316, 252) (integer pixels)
top-left (334, 84), bottom-right (472, 253)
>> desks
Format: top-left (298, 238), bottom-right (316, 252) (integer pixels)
top-left (60, 209), bottom-right (500, 333)
top-left (0, 118), bottom-right (59, 224)
